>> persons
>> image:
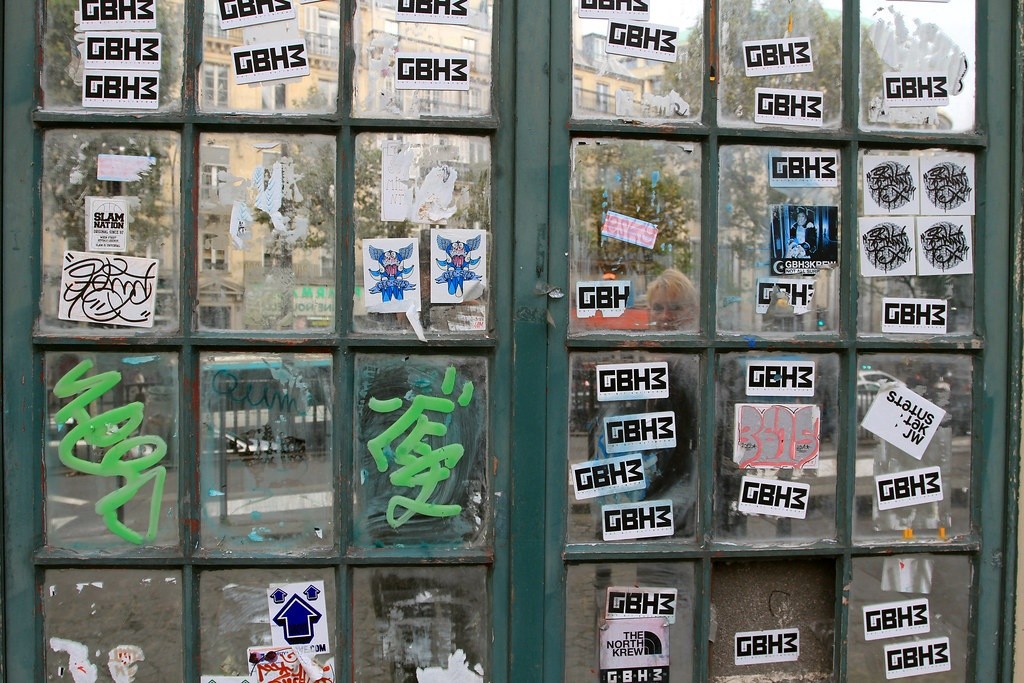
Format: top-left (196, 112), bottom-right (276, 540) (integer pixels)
top-left (354, 225), bottom-right (837, 682)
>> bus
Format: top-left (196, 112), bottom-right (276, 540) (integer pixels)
top-left (123, 355), bottom-right (332, 458)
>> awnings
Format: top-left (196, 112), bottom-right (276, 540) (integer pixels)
top-left (294, 354), bottom-right (333, 368)
top-left (202, 355), bottom-right (283, 370)
